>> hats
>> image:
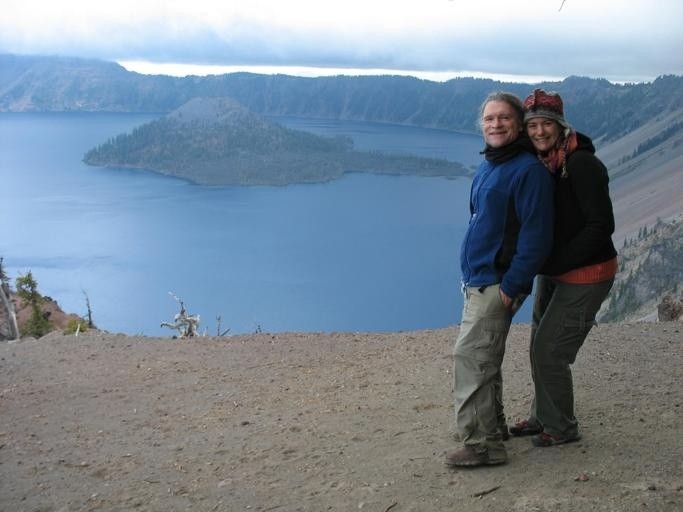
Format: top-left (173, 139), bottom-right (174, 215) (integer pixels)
top-left (523, 89), bottom-right (563, 122)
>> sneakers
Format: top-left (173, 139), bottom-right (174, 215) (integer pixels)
top-left (510, 420), bottom-right (542, 435)
top-left (532, 433), bottom-right (581, 447)
top-left (445, 445), bottom-right (504, 466)
top-left (453, 429), bottom-right (508, 443)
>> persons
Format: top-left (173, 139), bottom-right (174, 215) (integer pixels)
top-left (508, 88), bottom-right (620, 448)
top-left (444, 91), bottom-right (554, 467)
